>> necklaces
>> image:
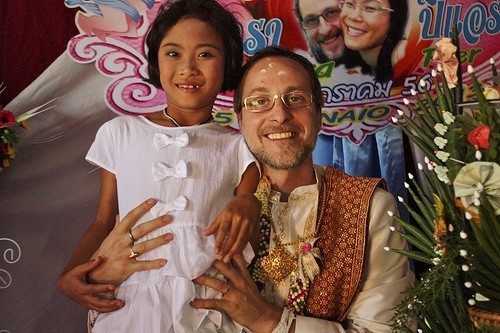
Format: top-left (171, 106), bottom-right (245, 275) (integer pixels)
top-left (163, 107), bottom-right (215, 127)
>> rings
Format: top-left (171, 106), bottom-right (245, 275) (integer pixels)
top-left (129, 246), bottom-right (139, 260)
top-left (128, 229), bottom-right (137, 242)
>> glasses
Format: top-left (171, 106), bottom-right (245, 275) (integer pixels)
top-left (301, 8), bottom-right (340, 30)
top-left (241, 91), bottom-right (317, 113)
top-left (339, 0), bottom-right (394, 15)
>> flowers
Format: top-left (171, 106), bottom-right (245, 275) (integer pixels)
top-left (383, 21), bottom-right (500, 333)
top-left (0, 84), bottom-right (60, 173)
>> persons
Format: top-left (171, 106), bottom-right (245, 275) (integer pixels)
top-left (296, 1), bottom-right (426, 80)
top-left (84, 45), bottom-right (425, 333)
top-left (55, 0), bottom-right (265, 333)
